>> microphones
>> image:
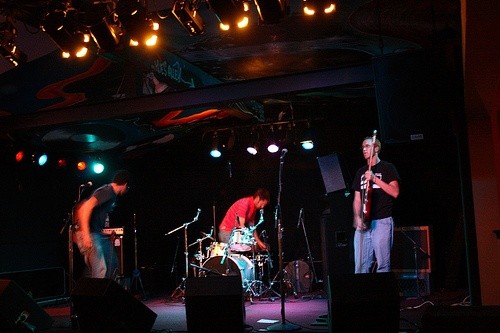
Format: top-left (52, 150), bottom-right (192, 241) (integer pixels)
top-left (280, 148), bottom-right (289, 159)
top-left (80, 181), bottom-right (93, 188)
top-left (297, 209), bottom-right (302, 228)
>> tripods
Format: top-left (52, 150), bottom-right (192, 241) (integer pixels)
top-left (242, 157), bottom-right (298, 304)
top-left (164, 214), bottom-right (215, 302)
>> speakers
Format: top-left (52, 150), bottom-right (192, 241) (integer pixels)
top-left (184, 274), bottom-right (246, 333)
top-left (0, 278), bottom-right (56, 333)
top-left (67, 276), bottom-right (157, 332)
top-left (327, 271), bottom-right (401, 333)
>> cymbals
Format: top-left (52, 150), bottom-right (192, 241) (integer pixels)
top-left (199, 231), bottom-right (217, 241)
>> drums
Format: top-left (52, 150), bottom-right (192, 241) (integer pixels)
top-left (229, 227), bottom-right (255, 252)
top-left (249, 254), bottom-right (269, 263)
top-left (209, 241), bottom-right (228, 258)
top-left (199, 255), bottom-right (255, 295)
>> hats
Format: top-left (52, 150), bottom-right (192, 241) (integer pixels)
top-left (113, 170), bottom-right (135, 188)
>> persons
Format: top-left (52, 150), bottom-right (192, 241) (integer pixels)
top-left (351, 136), bottom-right (400, 274)
top-left (72, 177), bottom-right (130, 280)
top-left (218, 189), bottom-right (272, 300)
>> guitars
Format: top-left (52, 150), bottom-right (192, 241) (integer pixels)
top-left (362, 130), bottom-right (377, 226)
